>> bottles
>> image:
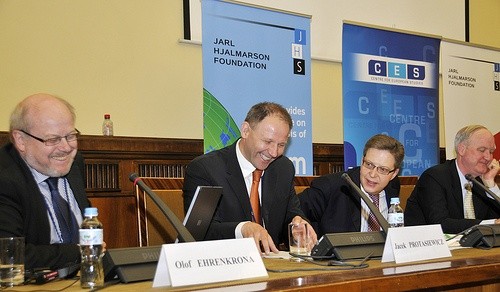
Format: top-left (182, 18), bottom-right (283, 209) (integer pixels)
top-left (102, 114), bottom-right (113, 136)
top-left (388, 197), bottom-right (404, 228)
top-left (79, 207), bottom-right (105, 288)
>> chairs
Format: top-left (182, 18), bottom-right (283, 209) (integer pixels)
top-left (133, 176), bottom-right (421, 248)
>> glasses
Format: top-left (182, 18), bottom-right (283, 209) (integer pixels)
top-left (362, 160), bottom-right (395, 175)
top-left (17, 129), bottom-right (80, 146)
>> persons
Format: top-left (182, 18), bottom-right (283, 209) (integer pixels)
top-left (177, 102), bottom-right (318, 256)
top-left (295, 135), bottom-right (405, 240)
top-left (403, 125), bottom-right (500, 235)
top-left (0, 94), bottom-right (107, 276)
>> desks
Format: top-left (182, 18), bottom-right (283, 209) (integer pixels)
top-left (0, 233), bottom-right (500, 292)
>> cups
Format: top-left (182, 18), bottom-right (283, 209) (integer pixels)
top-left (0, 237), bottom-right (24, 288)
top-left (288, 223), bottom-right (308, 261)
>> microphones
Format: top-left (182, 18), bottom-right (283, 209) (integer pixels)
top-left (465, 174), bottom-right (500, 203)
top-left (129, 173), bottom-right (197, 243)
top-left (341, 173), bottom-right (391, 234)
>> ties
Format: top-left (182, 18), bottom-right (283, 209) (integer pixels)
top-left (43, 177), bottom-right (80, 244)
top-left (368, 194), bottom-right (380, 232)
top-left (250, 170), bottom-right (262, 227)
top-left (464, 183), bottom-right (475, 219)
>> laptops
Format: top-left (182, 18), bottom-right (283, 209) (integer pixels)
top-left (175, 186), bottom-right (223, 244)
